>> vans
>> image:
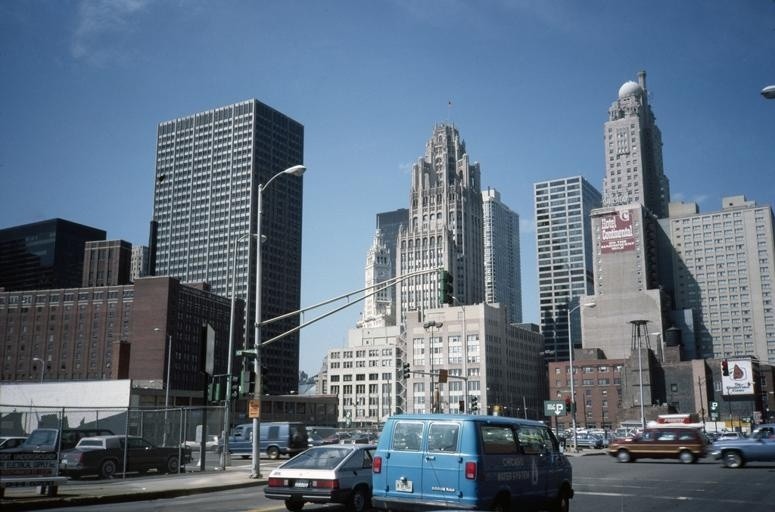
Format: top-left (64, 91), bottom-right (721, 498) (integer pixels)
top-left (216, 420), bottom-right (309, 461)
top-left (371, 414), bottom-right (574, 511)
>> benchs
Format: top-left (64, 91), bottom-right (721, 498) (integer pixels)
top-left (0, 450), bottom-right (68, 497)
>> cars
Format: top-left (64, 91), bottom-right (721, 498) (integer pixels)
top-left (181, 434), bottom-right (219, 452)
top-left (263, 444), bottom-right (377, 511)
top-left (556, 427), bottom-right (605, 449)
top-left (0, 427), bottom-right (192, 477)
top-left (604, 423), bottom-right (775, 470)
top-left (309, 429), bottom-right (378, 444)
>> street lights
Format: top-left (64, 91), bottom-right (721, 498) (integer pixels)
top-left (403, 296), bottom-right (469, 414)
top-left (567, 302), bottom-right (598, 428)
top-left (154, 327), bottom-right (172, 448)
top-left (32, 357), bottom-right (45, 384)
top-left (249, 163), bottom-right (444, 478)
